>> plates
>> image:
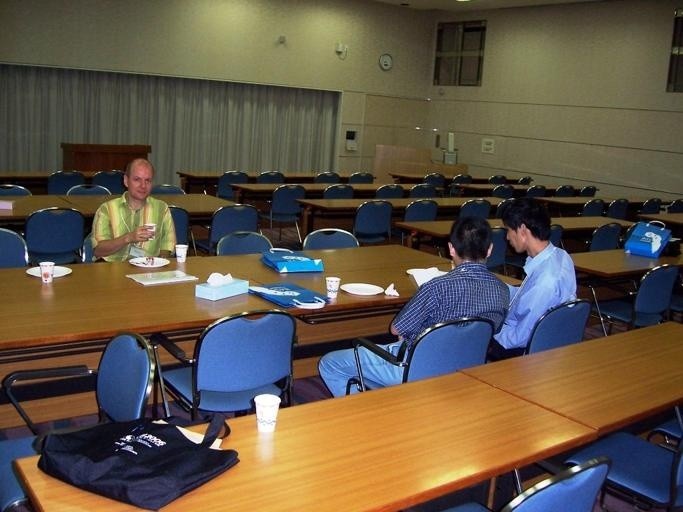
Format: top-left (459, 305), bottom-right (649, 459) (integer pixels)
top-left (340, 283), bottom-right (385, 296)
top-left (128, 256), bottom-right (170, 268)
top-left (25, 266), bottom-right (72, 277)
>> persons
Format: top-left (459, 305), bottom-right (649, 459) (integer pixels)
top-left (319, 216), bottom-right (510, 398)
top-left (91, 159), bottom-right (176, 263)
top-left (485, 204), bottom-right (576, 362)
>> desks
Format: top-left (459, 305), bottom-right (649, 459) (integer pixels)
top-left (389, 216), bottom-right (640, 251)
top-left (59, 193), bottom-right (241, 224)
top-left (534, 196), bottom-right (646, 217)
top-left (638, 213), bottom-right (683, 225)
top-left (454, 182), bottom-right (534, 197)
top-left (0, 194), bottom-right (93, 231)
top-left (297, 196), bottom-right (505, 233)
top-left (11, 370), bottom-right (600, 512)
top-left (0, 281), bottom-right (298, 405)
top-left (228, 182), bottom-right (448, 203)
top-left (62, 142), bottom-right (151, 170)
top-left (461, 320), bottom-right (683, 435)
top-left (202, 244), bottom-right (457, 281)
top-left (389, 173), bottom-right (511, 182)
top-left (0, 256), bottom-right (243, 298)
top-left (569, 243), bottom-right (683, 336)
top-left (245, 263), bottom-right (522, 360)
top-left (176, 171), bottom-right (379, 188)
top-left (0, 170), bottom-right (124, 193)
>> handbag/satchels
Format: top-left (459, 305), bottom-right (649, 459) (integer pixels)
top-left (626, 220), bottom-right (671, 259)
top-left (35, 410), bottom-right (242, 508)
top-left (261, 245), bottom-right (324, 272)
top-left (246, 282), bottom-right (330, 310)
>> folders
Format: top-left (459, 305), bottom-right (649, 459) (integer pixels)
top-left (124, 270), bottom-right (199, 287)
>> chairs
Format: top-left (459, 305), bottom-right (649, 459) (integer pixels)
top-left (448, 175), bottom-right (473, 198)
top-left (217, 230), bottom-right (274, 254)
top-left (487, 174), bottom-right (507, 183)
top-left (647, 413), bottom-right (683, 450)
top-left (65, 185), bottom-right (113, 197)
top-left (168, 206), bottom-right (189, 257)
top-left (0, 332), bottom-right (154, 511)
top-left (189, 204), bottom-right (260, 254)
top-left (525, 299), bottom-right (592, 355)
top-left (564, 430), bottom-right (683, 512)
top-left (519, 176), bottom-right (533, 186)
top-left (151, 185), bottom-right (187, 195)
top-left (216, 170), bottom-right (250, 202)
top-left (594, 263), bottom-right (679, 338)
top-left (505, 224), bottom-right (564, 283)
top-left (155, 309), bottom-right (297, 420)
top-left (431, 456), bottom-right (606, 512)
top-left (620, 219), bottom-right (641, 250)
top-left (256, 171), bottom-right (286, 182)
top-left (83, 232), bottom-right (94, 263)
top-left (410, 184), bottom-right (440, 199)
top-left (491, 184), bottom-right (517, 198)
top-left (667, 199), bottom-right (683, 214)
top-left (576, 199), bottom-right (604, 215)
top-left (486, 227), bottom-right (506, 275)
top-left (554, 185), bottom-right (577, 197)
top-left (393, 200), bottom-right (440, 246)
top-left (348, 200), bottom-right (393, 242)
top-left (314, 171), bottom-right (342, 182)
top-left (666, 262), bottom-right (683, 322)
top-left (91, 169), bottom-right (131, 196)
top-left (47, 169), bottom-right (86, 195)
top-left (524, 185), bottom-right (548, 198)
top-left (308, 183), bottom-right (353, 232)
top-left (580, 185), bottom-right (598, 196)
top-left (302, 228), bottom-right (362, 250)
top-left (0, 227), bottom-right (28, 267)
top-left (21, 207), bottom-right (86, 264)
top-left (376, 183), bottom-right (404, 198)
top-left (641, 198), bottom-right (661, 214)
top-left (606, 198), bottom-right (631, 218)
top-left (348, 171), bottom-right (376, 182)
top-left (346, 316), bottom-right (494, 394)
top-left (0, 182), bottom-right (31, 196)
top-left (577, 222), bottom-right (622, 289)
top-left (424, 173), bottom-right (447, 188)
top-left (259, 183), bottom-right (307, 247)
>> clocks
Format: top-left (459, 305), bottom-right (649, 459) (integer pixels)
top-left (380, 53), bottom-right (393, 70)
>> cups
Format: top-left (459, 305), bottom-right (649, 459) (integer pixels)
top-left (175, 245), bottom-right (188, 262)
top-left (144, 224), bottom-right (156, 240)
top-left (325, 277), bottom-right (341, 299)
top-left (40, 262), bottom-right (55, 283)
top-left (254, 394), bottom-right (282, 433)
